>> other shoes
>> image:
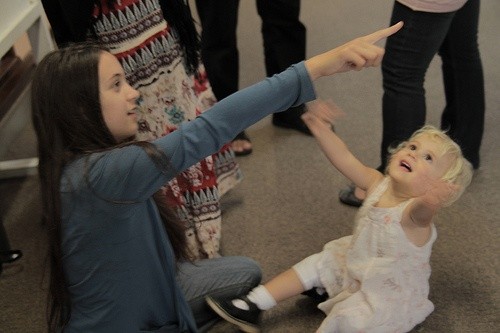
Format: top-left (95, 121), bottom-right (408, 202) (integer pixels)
top-left (231, 132), bottom-right (253, 156)
top-left (340, 186), bottom-right (363, 206)
top-left (273, 104), bottom-right (313, 137)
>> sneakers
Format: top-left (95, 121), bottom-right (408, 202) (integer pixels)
top-left (205, 294), bottom-right (266, 333)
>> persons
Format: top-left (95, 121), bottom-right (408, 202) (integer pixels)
top-left (204, 110), bottom-right (473, 333)
top-left (339, 0), bottom-right (485, 207)
top-left (31, 0), bottom-right (404, 333)
